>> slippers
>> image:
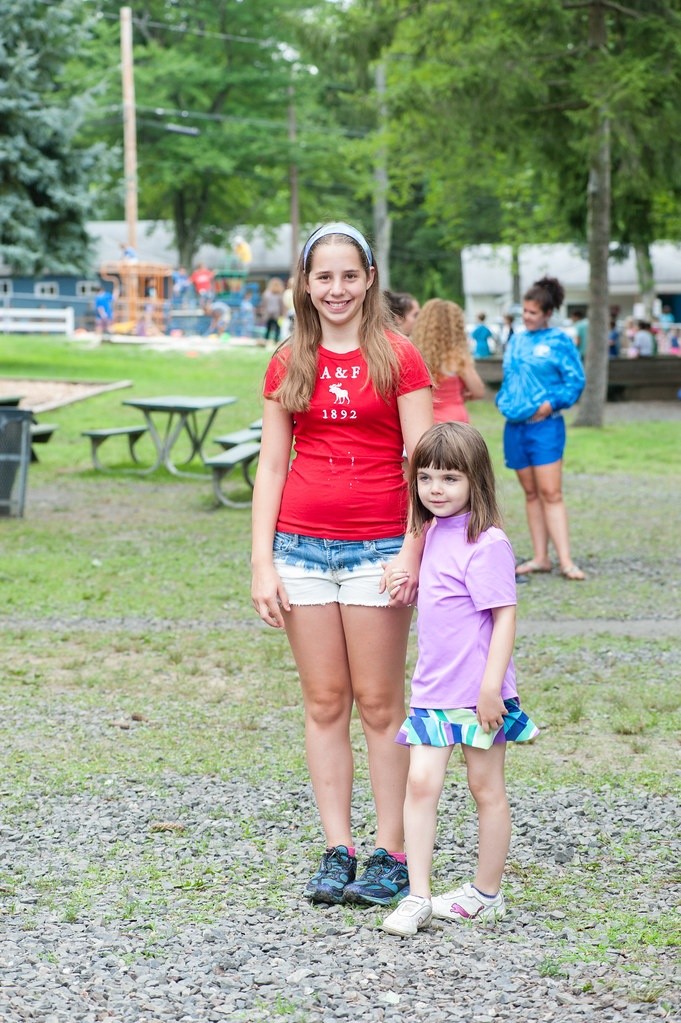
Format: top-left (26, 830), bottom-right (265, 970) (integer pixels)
top-left (560, 565), bottom-right (585, 581)
top-left (516, 560), bottom-right (551, 575)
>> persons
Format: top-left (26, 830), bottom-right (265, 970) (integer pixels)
top-left (202, 300), bottom-right (232, 338)
top-left (494, 277), bottom-right (588, 580)
top-left (240, 289), bottom-right (257, 339)
top-left (409, 297), bottom-right (484, 425)
top-left (571, 308), bottom-right (589, 361)
top-left (608, 299), bottom-right (681, 359)
top-left (171, 263), bottom-right (219, 310)
top-left (378, 421), bottom-right (541, 935)
top-left (251, 218), bottom-right (437, 903)
top-left (499, 316), bottom-right (515, 352)
top-left (258, 274), bottom-right (297, 349)
top-left (470, 313), bottom-right (499, 357)
top-left (91, 282), bottom-right (116, 336)
top-left (118, 242), bottom-right (138, 264)
top-left (382, 289), bottom-right (419, 337)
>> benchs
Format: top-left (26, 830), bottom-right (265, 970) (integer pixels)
top-left (204, 420), bottom-right (265, 509)
top-left (82, 427), bottom-right (145, 474)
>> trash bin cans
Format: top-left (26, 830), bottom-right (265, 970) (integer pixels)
top-left (0, 407), bottom-right (38, 516)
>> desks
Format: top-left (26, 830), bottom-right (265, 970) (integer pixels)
top-left (122, 395), bottom-right (236, 480)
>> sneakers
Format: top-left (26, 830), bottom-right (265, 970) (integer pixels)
top-left (343, 848), bottom-right (410, 906)
top-left (431, 883), bottom-right (506, 922)
top-left (381, 895), bottom-right (432, 937)
top-left (303, 845), bottom-right (357, 904)
